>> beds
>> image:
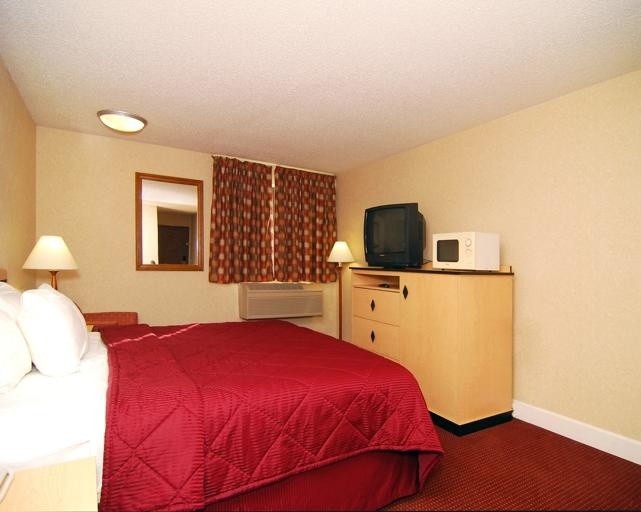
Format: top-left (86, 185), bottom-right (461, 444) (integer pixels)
top-left (0, 265), bottom-right (423, 512)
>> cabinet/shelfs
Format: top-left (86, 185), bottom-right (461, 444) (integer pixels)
top-left (351, 268), bottom-right (513, 426)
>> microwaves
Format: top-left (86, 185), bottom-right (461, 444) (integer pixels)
top-left (432, 233), bottom-right (500, 271)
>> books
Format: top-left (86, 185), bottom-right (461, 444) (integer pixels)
top-left (349, 266), bottom-right (384, 269)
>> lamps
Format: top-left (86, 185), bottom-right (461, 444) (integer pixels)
top-left (21, 234), bottom-right (79, 291)
top-left (95, 107), bottom-right (147, 135)
top-left (324, 240), bottom-right (355, 339)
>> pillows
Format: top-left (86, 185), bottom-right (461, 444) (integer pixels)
top-left (0, 281), bottom-right (91, 390)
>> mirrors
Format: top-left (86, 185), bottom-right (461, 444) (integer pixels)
top-left (134, 172), bottom-right (205, 271)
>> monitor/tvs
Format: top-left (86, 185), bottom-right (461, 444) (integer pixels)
top-left (363, 202), bottom-right (427, 271)
